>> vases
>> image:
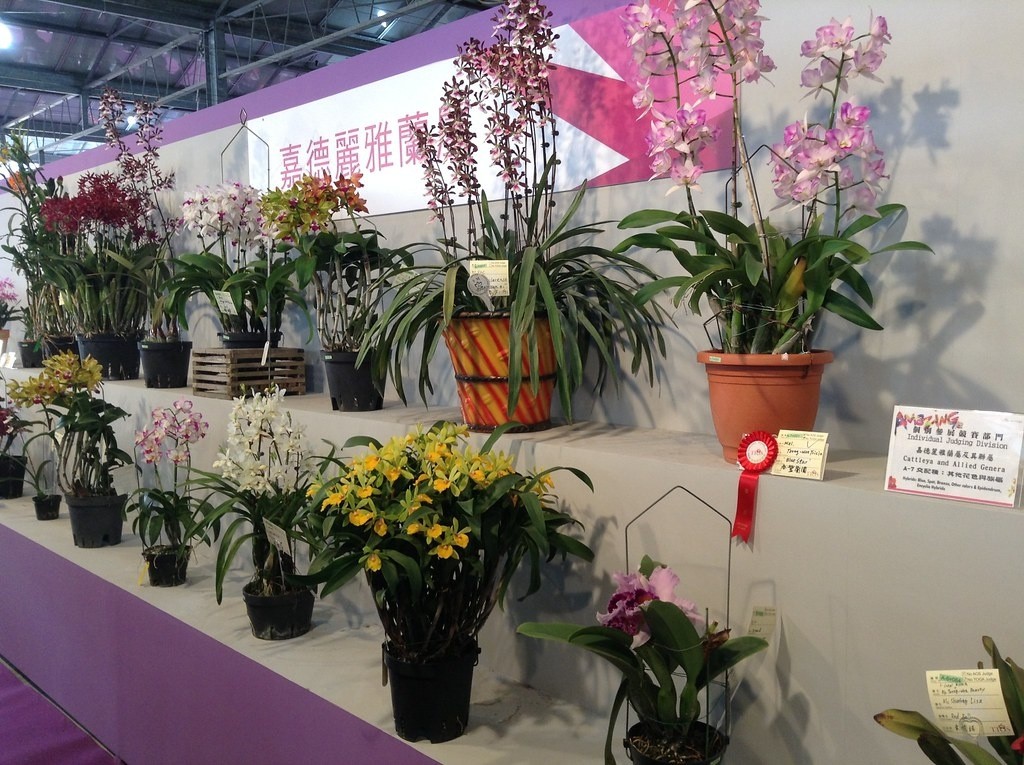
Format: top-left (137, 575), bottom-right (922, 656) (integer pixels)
top-left (321, 346), bottom-right (391, 414)
top-left (137, 341), bottom-right (193, 388)
top-left (65, 489), bottom-right (129, 548)
top-left (242, 576), bottom-right (315, 641)
top-left (0, 330), bottom-right (9, 353)
top-left (381, 642), bottom-right (481, 745)
top-left (218, 333), bottom-right (280, 398)
top-left (142, 544), bottom-right (192, 586)
top-left (77, 336), bottom-right (125, 381)
top-left (438, 313), bottom-right (560, 434)
top-left (695, 349), bottom-right (834, 464)
top-left (45, 342), bottom-right (79, 365)
top-left (0, 456), bottom-right (28, 499)
top-left (623, 721), bottom-right (729, 765)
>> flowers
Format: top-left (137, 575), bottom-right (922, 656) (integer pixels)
top-left (257, 167), bottom-right (413, 351)
top-left (292, 420), bottom-right (595, 658)
top-left (153, 179), bottom-right (313, 342)
top-left (0, 277), bottom-right (23, 328)
top-left (5, 349), bottom-right (135, 497)
top-left (122, 395), bottom-right (221, 569)
top-left (88, 88), bottom-right (220, 342)
top-left (355, 0), bottom-right (678, 426)
top-left (610, 0), bottom-right (936, 356)
top-left (0, 123), bottom-right (81, 337)
top-left (515, 553), bottom-right (768, 765)
top-left (0, 400), bottom-right (33, 455)
top-left (175, 384), bottom-right (337, 606)
top-left (40, 173), bottom-right (144, 336)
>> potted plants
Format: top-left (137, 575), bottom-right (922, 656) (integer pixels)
top-left (0, 457), bottom-right (62, 521)
top-left (16, 305), bottom-right (42, 368)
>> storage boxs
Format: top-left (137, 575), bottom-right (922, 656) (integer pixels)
top-left (192, 347), bottom-right (304, 400)
